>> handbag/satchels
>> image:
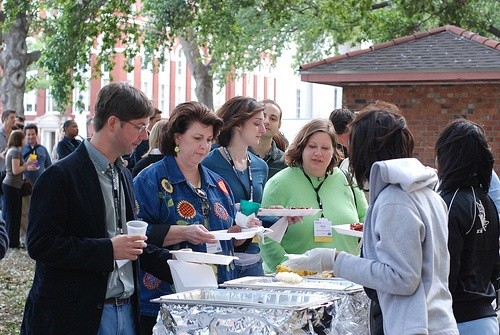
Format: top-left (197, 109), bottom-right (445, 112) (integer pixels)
top-left (21, 177), bottom-right (33, 197)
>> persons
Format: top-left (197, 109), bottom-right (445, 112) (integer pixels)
top-left (249, 98), bottom-right (288, 182)
top-left (19, 81), bottom-right (205, 335)
top-left (132, 100), bottom-right (260, 335)
top-left (256, 117), bottom-right (369, 278)
top-left (121, 107), bottom-right (168, 177)
top-left (329, 107), bottom-right (370, 206)
top-left (432, 117), bottom-right (500, 335)
top-left (278, 100), bottom-right (459, 335)
top-left (0, 110), bottom-right (64, 259)
top-left (200, 96), bottom-right (305, 289)
top-left (56, 119), bottom-right (83, 161)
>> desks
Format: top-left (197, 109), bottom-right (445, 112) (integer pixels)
top-left (148, 273), bottom-right (371, 335)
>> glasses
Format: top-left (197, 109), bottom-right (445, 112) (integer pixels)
top-left (123, 120), bottom-right (150, 134)
top-left (195, 188), bottom-right (212, 218)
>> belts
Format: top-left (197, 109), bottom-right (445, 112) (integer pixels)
top-left (106, 297), bottom-right (133, 306)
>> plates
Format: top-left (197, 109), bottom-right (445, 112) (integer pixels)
top-left (168, 250), bottom-right (240, 265)
top-left (331, 223), bottom-right (363, 238)
top-left (209, 227), bottom-right (261, 240)
top-left (259, 208), bottom-right (322, 216)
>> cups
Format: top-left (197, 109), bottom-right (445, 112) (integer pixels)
top-left (239, 199), bottom-right (260, 218)
top-left (30, 154), bottom-right (37, 160)
top-left (126, 220), bottom-right (148, 242)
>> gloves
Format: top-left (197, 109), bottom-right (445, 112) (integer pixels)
top-left (281, 248), bottom-right (336, 273)
